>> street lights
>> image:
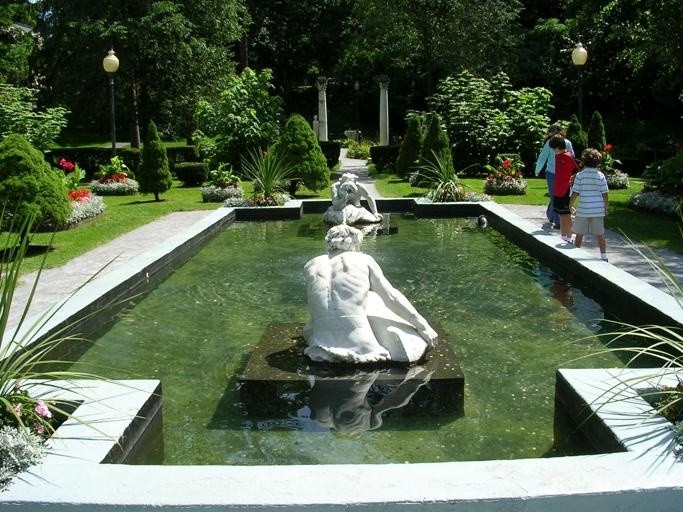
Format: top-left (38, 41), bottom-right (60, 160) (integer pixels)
top-left (102, 45), bottom-right (120, 156)
top-left (571, 39), bottom-right (588, 121)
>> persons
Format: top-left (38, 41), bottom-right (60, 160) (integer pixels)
top-left (535, 124), bottom-right (576, 229)
top-left (549, 133), bottom-right (580, 243)
top-left (303, 225), bottom-right (439, 367)
top-left (323, 172), bottom-right (385, 226)
top-left (569, 148), bottom-right (609, 262)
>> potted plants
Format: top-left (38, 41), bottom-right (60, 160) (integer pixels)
top-left (403, 150), bottom-right (472, 202)
top-left (201, 146), bottom-right (293, 206)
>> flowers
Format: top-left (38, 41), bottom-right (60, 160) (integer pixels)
top-left (48, 153), bottom-right (140, 224)
top-left (485, 150), bottom-right (527, 193)
top-left (0, 372), bottom-right (55, 492)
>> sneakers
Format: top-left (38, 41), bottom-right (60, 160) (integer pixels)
top-left (549, 220), bottom-right (560, 229)
top-left (602, 258), bottom-right (609, 263)
